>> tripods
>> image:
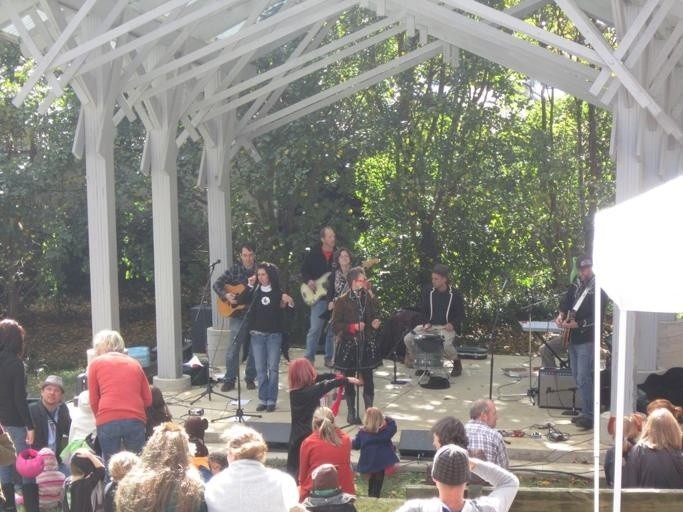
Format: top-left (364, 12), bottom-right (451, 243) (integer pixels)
top-left (211, 295), bottom-right (262, 423)
top-left (190, 268), bottom-right (238, 405)
top-left (511, 300), bottom-right (540, 405)
top-left (340, 313), bottom-right (365, 430)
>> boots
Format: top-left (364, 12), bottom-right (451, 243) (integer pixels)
top-left (3, 483), bottom-right (17, 512)
top-left (23, 484), bottom-right (40, 512)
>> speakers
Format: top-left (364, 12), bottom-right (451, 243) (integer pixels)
top-left (191, 304), bottom-right (212, 353)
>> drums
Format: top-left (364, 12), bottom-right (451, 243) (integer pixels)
top-left (413, 333), bottom-right (442, 354)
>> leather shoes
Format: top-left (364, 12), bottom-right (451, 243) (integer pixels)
top-left (256, 404), bottom-right (267, 411)
top-left (247, 381), bottom-right (255, 390)
top-left (572, 415), bottom-right (583, 423)
top-left (451, 358), bottom-right (462, 376)
top-left (221, 382), bottom-right (234, 391)
top-left (577, 417), bottom-right (594, 428)
top-left (268, 404), bottom-right (275, 411)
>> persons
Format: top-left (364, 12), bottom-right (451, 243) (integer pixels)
top-left (648, 399), bottom-right (680, 418)
top-left (405, 266), bottom-right (464, 378)
top-left (103, 450), bottom-right (140, 512)
top-left (331, 267), bottom-right (385, 424)
top-left (27, 375), bottom-right (71, 477)
top-left (87, 330), bottom-right (152, 484)
top-left (1, 317), bottom-right (39, 511)
top-left (285, 357), bottom-right (361, 486)
top-left (1, 424), bottom-right (19, 511)
top-left (298, 406), bottom-right (358, 503)
top-left (394, 444), bottom-right (519, 512)
top-left (464, 398), bottom-right (509, 469)
top-left (213, 240), bottom-right (259, 391)
top-left (198, 450), bottom-right (229, 483)
top-left (328, 247), bottom-right (353, 310)
top-left (115, 422), bottom-right (207, 512)
top-left (145, 386), bottom-right (172, 441)
top-left (621, 408), bottom-right (683, 491)
top-left (300, 226), bottom-right (340, 369)
top-left (350, 406), bottom-right (399, 498)
top-left (426, 416), bottom-right (491, 488)
top-left (557, 253), bottom-right (604, 428)
top-left (63, 448), bottom-right (106, 512)
top-left (540, 336), bottom-right (570, 368)
top-left (301, 464), bottom-right (357, 512)
top-left (605, 417), bottom-right (636, 485)
top-left (205, 423), bottom-right (308, 512)
top-left (185, 417), bottom-right (209, 458)
top-left (36, 447), bottom-right (67, 511)
top-left (236, 262), bottom-right (294, 412)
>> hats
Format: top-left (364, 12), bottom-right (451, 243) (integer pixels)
top-left (429, 264), bottom-right (449, 275)
top-left (310, 465), bottom-right (338, 488)
top-left (432, 445), bottom-right (471, 484)
top-left (41, 376), bottom-right (64, 393)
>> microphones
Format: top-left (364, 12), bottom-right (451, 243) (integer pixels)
top-left (208, 259), bottom-right (222, 268)
top-left (252, 282), bottom-right (262, 294)
top-left (349, 288), bottom-right (368, 314)
top-left (502, 277), bottom-right (510, 291)
top-left (526, 288), bottom-right (532, 298)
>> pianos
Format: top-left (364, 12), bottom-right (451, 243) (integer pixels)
top-left (517, 321), bottom-right (564, 333)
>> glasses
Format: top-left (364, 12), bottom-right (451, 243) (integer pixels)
top-left (355, 277), bottom-right (367, 283)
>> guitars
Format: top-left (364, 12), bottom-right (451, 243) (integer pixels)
top-left (560, 309), bottom-right (577, 348)
top-left (216, 283), bottom-right (249, 318)
top-left (300, 271), bottom-right (333, 306)
